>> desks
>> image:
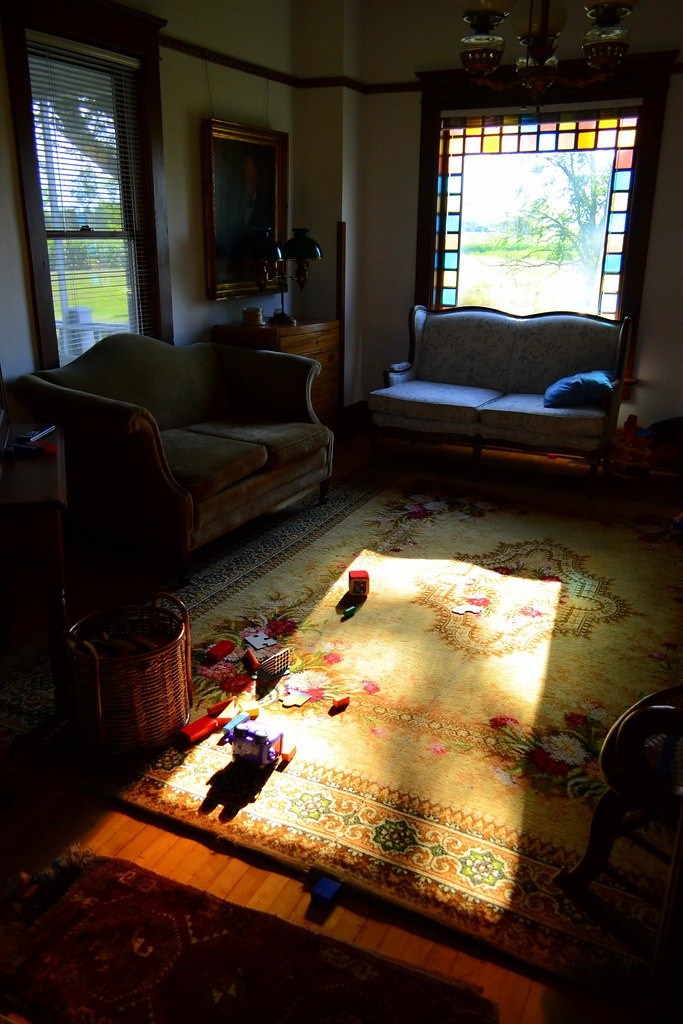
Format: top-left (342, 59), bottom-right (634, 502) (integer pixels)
top-left (0, 425), bottom-right (78, 720)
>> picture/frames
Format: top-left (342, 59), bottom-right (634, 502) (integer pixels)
top-left (194, 116), bottom-right (290, 302)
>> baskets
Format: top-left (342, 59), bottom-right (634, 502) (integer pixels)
top-left (62, 591), bottom-right (194, 756)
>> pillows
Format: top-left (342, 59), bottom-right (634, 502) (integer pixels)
top-left (543, 365), bottom-right (616, 406)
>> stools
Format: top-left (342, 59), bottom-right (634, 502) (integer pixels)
top-left (553, 686), bottom-right (682, 912)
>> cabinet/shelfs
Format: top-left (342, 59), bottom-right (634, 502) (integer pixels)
top-left (212, 315), bottom-right (346, 449)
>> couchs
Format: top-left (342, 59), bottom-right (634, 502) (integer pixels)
top-left (18, 331), bottom-right (336, 588)
top-left (365, 305), bottom-right (634, 498)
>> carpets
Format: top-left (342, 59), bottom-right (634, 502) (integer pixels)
top-left (1, 455), bottom-right (682, 1023)
top-left (1, 852), bottom-right (499, 1024)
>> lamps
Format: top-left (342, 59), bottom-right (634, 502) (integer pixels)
top-left (446, 0), bottom-right (633, 118)
top-left (259, 226), bottom-right (322, 327)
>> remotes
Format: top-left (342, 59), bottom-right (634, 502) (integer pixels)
top-left (15, 423), bottom-right (56, 445)
